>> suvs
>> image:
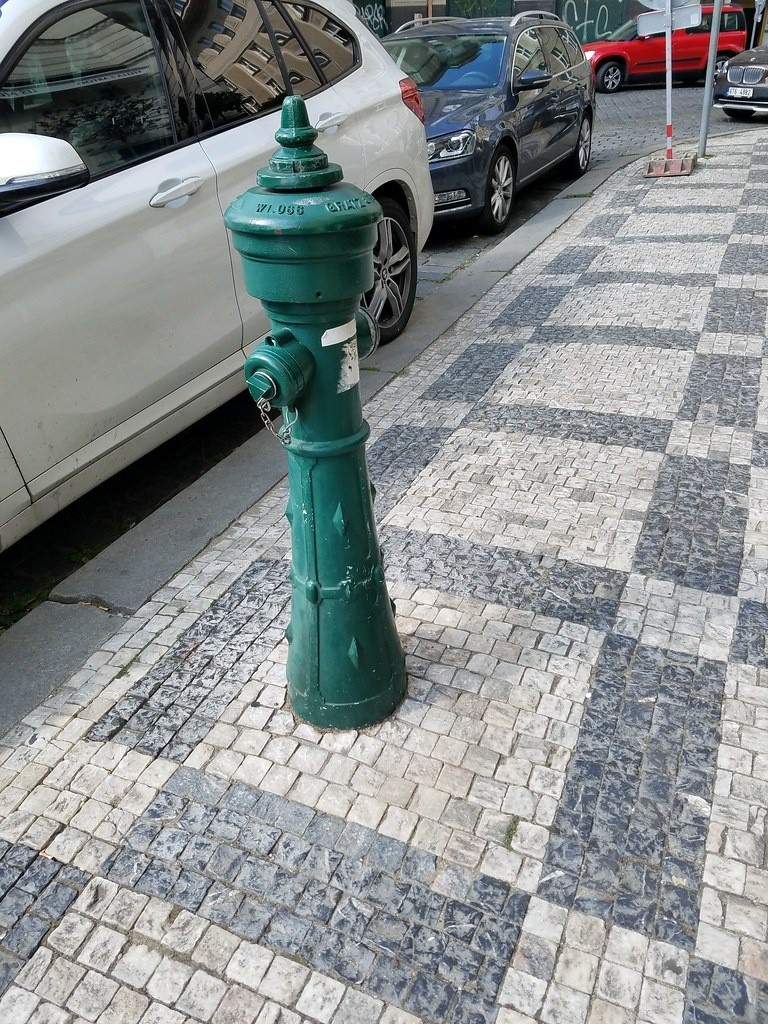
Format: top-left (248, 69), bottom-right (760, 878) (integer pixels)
top-left (380, 10), bottom-right (600, 238)
top-left (0, 2), bottom-right (437, 554)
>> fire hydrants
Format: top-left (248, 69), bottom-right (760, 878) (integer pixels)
top-left (222, 93), bottom-right (409, 734)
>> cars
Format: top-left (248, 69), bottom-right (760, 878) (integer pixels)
top-left (712, 40), bottom-right (768, 117)
top-left (581, 2), bottom-right (748, 93)
